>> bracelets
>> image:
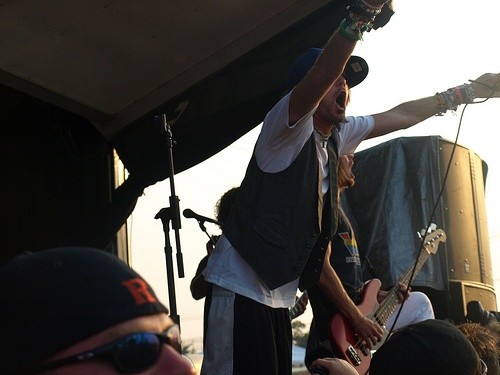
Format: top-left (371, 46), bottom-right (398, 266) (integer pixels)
top-left (339, 0), bottom-right (385, 40)
top-left (434, 82), bottom-right (476, 116)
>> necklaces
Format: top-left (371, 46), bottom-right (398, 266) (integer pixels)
top-left (314, 127), bottom-right (332, 149)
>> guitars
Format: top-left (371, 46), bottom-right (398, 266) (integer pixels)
top-left (289, 290), bottom-right (312, 321)
top-left (328, 221), bottom-right (448, 375)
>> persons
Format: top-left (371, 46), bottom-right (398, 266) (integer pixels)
top-left (311, 318), bottom-right (500, 375)
top-left (190, 0), bottom-right (500, 375)
top-left (0, 247), bottom-right (196, 375)
top-left (304, 153), bottom-right (412, 375)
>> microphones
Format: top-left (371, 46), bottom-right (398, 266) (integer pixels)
top-left (183, 209), bottom-right (220, 224)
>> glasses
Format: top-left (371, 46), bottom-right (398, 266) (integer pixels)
top-left (43, 322), bottom-right (184, 373)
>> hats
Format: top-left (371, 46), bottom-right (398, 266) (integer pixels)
top-left (368, 319), bottom-right (483, 375)
top-left (289, 48), bottom-right (369, 88)
top-left (0, 247), bottom-right (173, 375)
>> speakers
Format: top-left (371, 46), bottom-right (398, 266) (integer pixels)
top-left (340, 134), bottom-right (498, 327)
